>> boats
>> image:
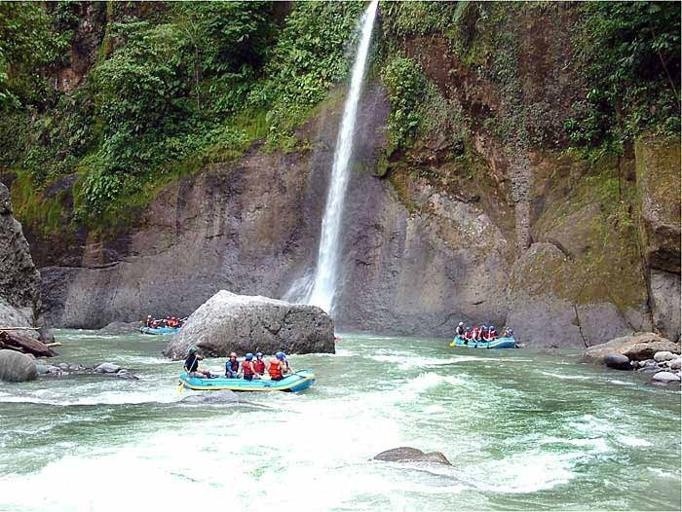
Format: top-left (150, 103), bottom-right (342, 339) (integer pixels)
top-left (179, 367), bottom-right (316, 393)
top-left (450, 334), bottom-right (516, 350)
top-left (138, 326), bottom-right (180, 335)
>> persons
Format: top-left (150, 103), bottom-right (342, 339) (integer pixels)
top-left (225, 351), bottom-right (242, 378)
top-left (144, 314), bottom-right (185, 330)
top-left (252, 352), bottom-right (267, 379)
top-left (453, 320), bottom-right (513, 345)
top-left (282, 354), bottom-right (294, 374)
top-left (240, 353), bottom-right (263, 379)
top-left (266, 352), bottom-right (287, 379)
top-left (184, 347), bottom-right (210, 378)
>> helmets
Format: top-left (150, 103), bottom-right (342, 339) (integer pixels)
top-left (465, 325), bottom-right (494, 333)
top-left (228, 350), bottom-right (287, 361)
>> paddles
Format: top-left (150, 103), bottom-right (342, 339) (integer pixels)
top-left (289, 372), bottom-right (314, 380)
top-left (178, 356), bottom-right (197, 389)
top-left (449, 326), bottom-right (491, 350)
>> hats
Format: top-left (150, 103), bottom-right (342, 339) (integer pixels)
top-left (459, 322), bottom-right (464, 326)
top-left (189, 346), bottom-right (197, 354)
